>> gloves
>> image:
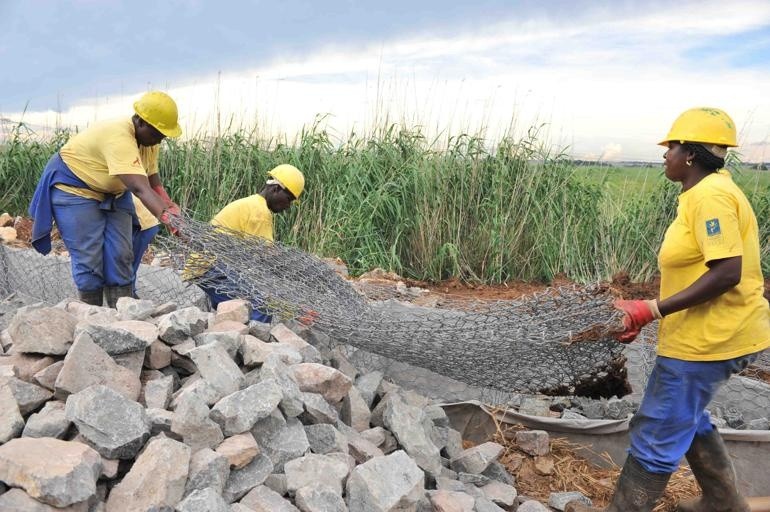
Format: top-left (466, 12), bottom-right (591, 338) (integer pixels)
top-left (613, 299), bottom-right (663, 344)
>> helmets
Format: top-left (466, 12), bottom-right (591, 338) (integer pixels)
top-left (133, 91), bottom-right (182, 138)
top-left (658, 108), bottom-right (737, 147)
top-left (267, 164), bottom-right (305, 200)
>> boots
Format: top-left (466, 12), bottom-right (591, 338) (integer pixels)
top-left (677, 424), bottom-right (752, 512)
top-left (565, 454), bottom-right (672, 512)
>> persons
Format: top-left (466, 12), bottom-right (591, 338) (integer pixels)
top-left (24, 90), bottom-right (195, 314)
top-left (180, 162), bottom-right (306, 325)
top-left (604, 104), bottom-right (770, 512)
top-left (130, 190), bottom-right (161, 297)
